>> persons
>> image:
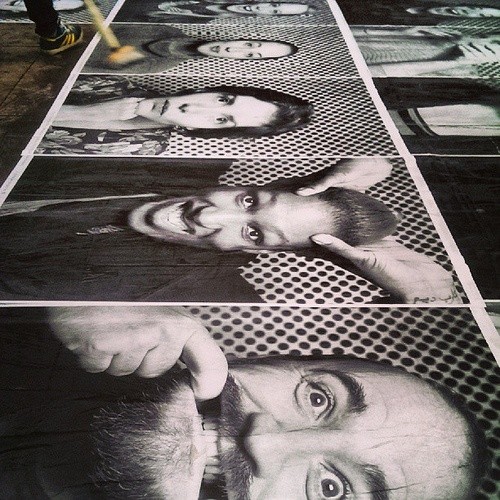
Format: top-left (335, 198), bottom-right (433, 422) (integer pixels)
top-left (112, 0), bottom-right (318, 23)
top-left (23, 0), bottom-right (86, 55)
top-left (80, 22), bottom-right (298, 74)
top-left (2, 304), bottom-right (486, 500)
top-left (336, 0), bottom-right (500, 26)
top-left (348, 25), bottom-right (500, 78)
top-left (33, 73), bottom-right (313, 156)
top-left (1, 157), bottom-right (462, 305)
top-left (372, 77), bottom-right (500, 155)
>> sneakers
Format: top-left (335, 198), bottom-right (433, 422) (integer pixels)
top-left (39, 18), bottom-right (83, 56)
